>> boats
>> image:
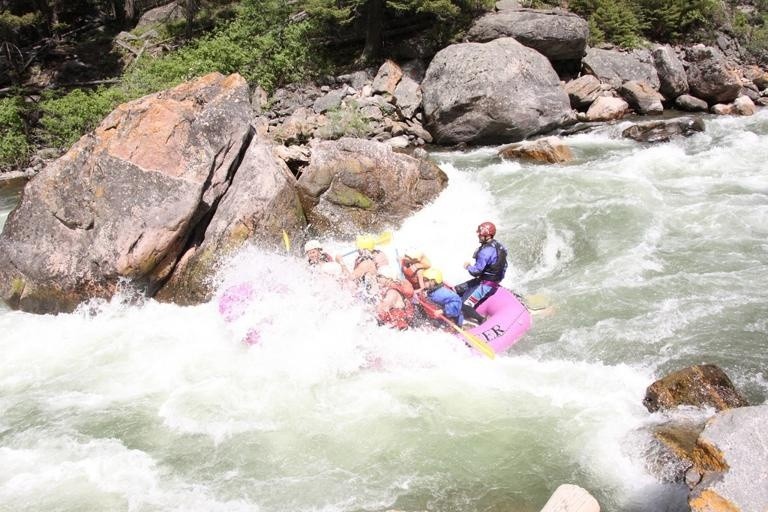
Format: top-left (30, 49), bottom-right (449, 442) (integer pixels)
top-left (218, 284), bottom-right (529, 373)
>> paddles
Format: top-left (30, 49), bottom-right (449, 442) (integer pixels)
top-left (416, 297), bottom-right (495, 359)
top-left (283, 230), bottom-right (290, 256)
top-left (341, 232), bottom-right (392, 258)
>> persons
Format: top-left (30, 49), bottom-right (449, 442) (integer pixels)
top-left (453, 220), bottom-right (508, 326)
top-left (292, 232), bottom-right (463, 336)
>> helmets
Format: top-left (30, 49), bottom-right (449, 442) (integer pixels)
top-left (376, 265), bottom-right (398, 282)
top-left (476, 222), bottom-right (496, 236)
top-left (404, 247), bottom-right (424, 262)
top-left (303, 239), bottom-right (323, 255)
top-left (356, 236), bottom-right (376, 250)
top-left (422, 267), bottom-right (443, 285)
